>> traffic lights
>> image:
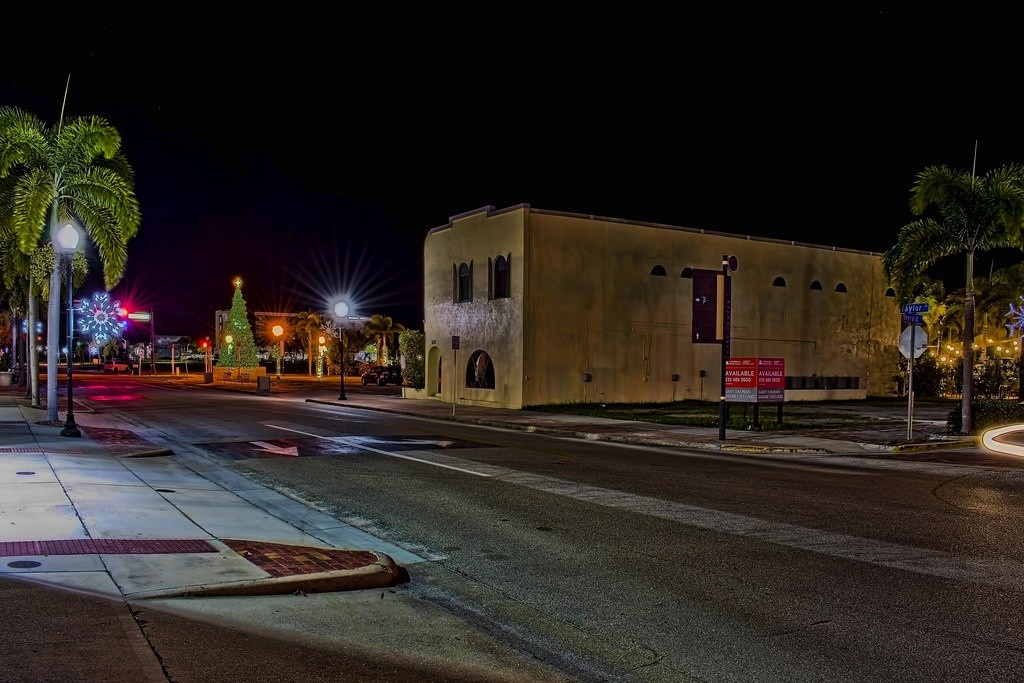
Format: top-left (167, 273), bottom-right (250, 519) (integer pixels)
top-left (203, 340), bottom-right (207, 348)
top-left (122, 310), bottom-right (127, 319)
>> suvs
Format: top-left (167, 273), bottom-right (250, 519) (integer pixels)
top-left (103, 360), bottom-right (129, 373)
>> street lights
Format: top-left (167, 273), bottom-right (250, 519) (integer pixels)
top-left (225, 335), bottom-right (241, 375)
top-left (272, 325), bottom-right (283, 381)
top-left (56, 223), bottom-right (82, 438)
top-left (333, 300), bottom-right (350, 402)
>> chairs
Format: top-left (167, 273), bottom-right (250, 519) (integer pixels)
top-left (233, 374), bottom-right (254, 391)
top-left (217, 372), bottom-right (233, 390)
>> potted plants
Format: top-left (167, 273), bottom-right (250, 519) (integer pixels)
top-left (0, 352), bottom-right (16, 386)
